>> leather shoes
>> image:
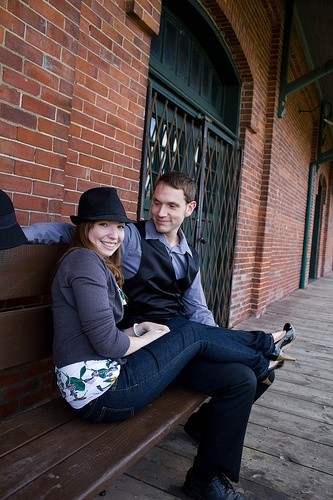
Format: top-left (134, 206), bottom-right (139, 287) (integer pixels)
top-left (181, 467), bottom-right (247, 500)
top-left (184, 417), bottom-right (199, 441)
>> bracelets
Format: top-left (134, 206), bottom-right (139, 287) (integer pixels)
top-left (133, 323), bottom-right (139, 337)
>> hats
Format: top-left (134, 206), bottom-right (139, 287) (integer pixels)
top-left (0, 189), bottom-right (29, 250)
top-left (69, 186), bottom-right (139, 225)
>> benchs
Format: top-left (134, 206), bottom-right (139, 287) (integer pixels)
top-left (0, 243), bottom-right (209, 500)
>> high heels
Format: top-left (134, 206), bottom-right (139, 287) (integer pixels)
top-left (269, 322), bottom-right (297, 361)
top-left (257, 359), bottom-right (284, 386)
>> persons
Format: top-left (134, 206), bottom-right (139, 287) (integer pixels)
top-left (51, 186), bottom-right (297, 420)
top-left (20, 170), bottom-right (276, 500)
